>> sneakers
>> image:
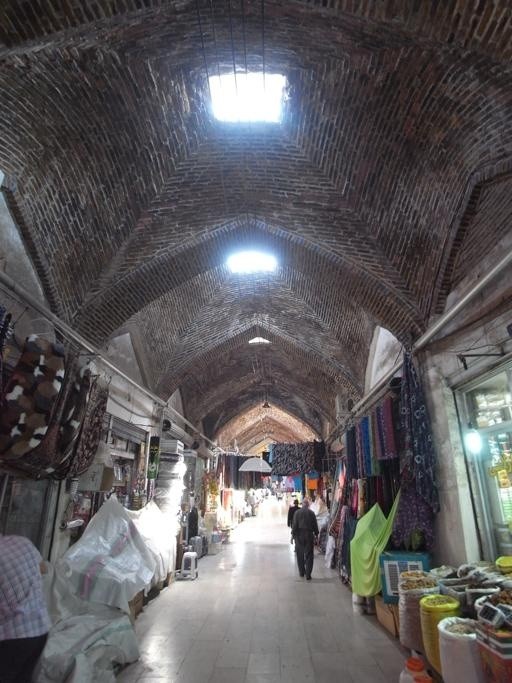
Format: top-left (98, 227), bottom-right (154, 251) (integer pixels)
top-left (306, 575), bottom-right (312, 580)
top-left (299, 573), bottom-right (304, 577)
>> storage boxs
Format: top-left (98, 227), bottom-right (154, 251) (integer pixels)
top-left (376, 546), bottom-right (433, 608)
top-left (472, 619), bottom-right (512, 683)
top-left (372, 591), bottom-right (400, 640)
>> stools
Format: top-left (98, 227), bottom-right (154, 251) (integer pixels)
top-left (180, 551), bottom-right (199, 580)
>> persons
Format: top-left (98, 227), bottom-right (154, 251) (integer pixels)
top-left (0, 533), bottom-right (51, 683)
top-left (290, 498), bottom-right (320, 580)
top-left (287, 499), bottom-right (302, 544)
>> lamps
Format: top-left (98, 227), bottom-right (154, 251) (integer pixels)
top-left (456, 350), bottom-right (504, 456)
top-left (262, 390), bottom-right (271, 408)
